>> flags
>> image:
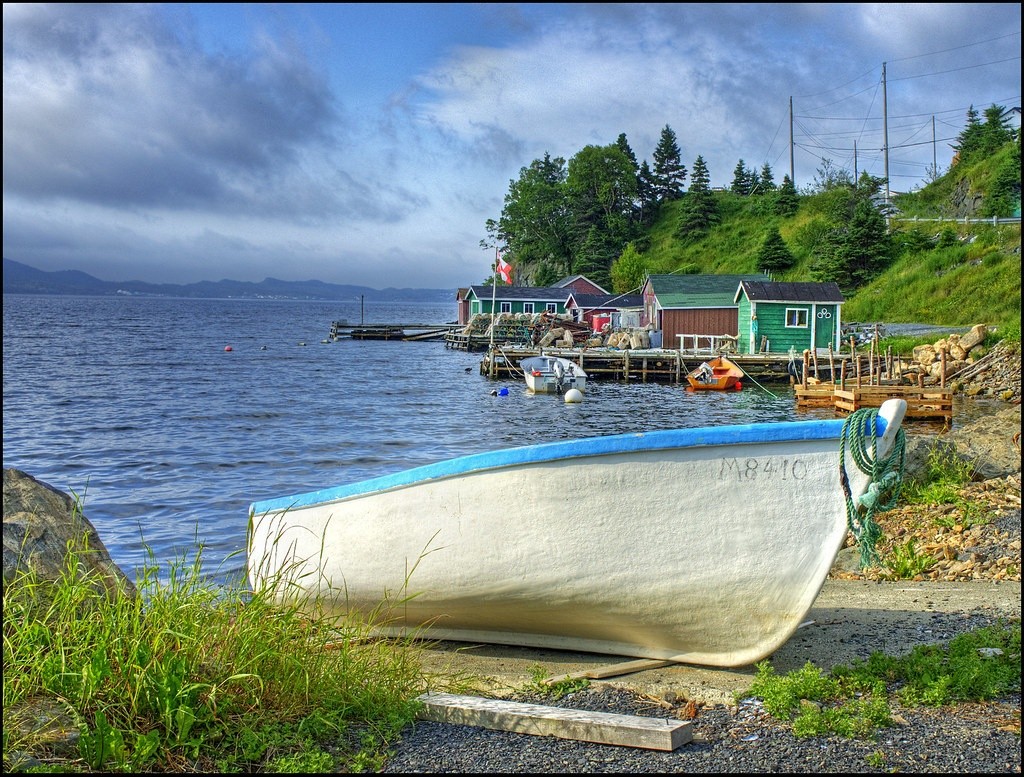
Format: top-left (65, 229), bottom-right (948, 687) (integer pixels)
top-left (496, 249), bottom-right (512, 284)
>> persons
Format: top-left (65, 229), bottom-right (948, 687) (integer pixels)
top-left (531, 325), bottom-right (542, 344)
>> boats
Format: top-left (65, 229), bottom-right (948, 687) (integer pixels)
top-left (519, 354), bottom-right (589, 395)
top-left (246, 397), bottom-right (908, 669)
top-left (685, 355), bottom-right (745, 392)
